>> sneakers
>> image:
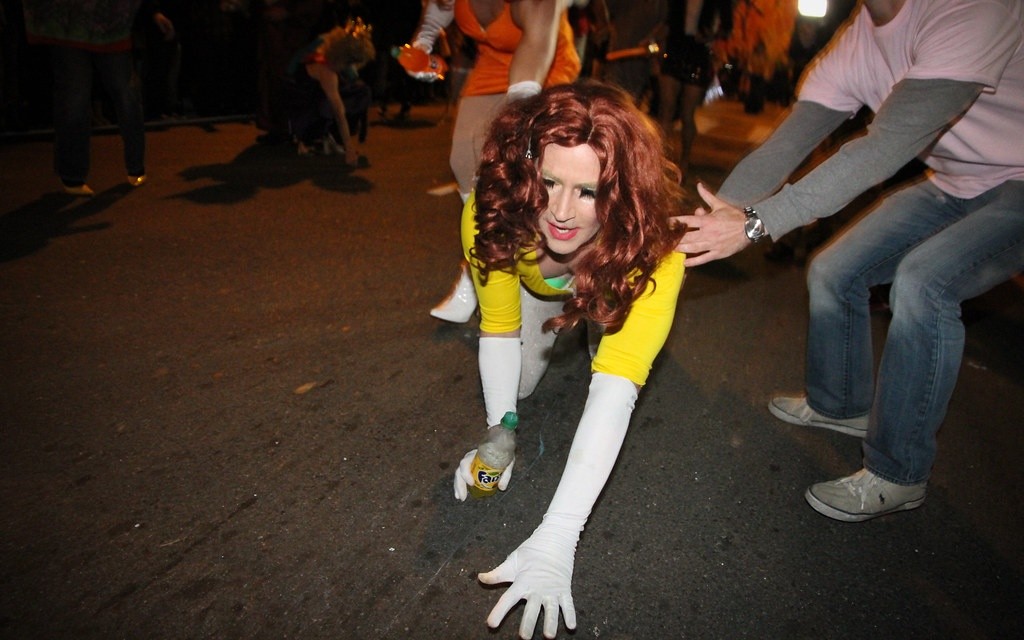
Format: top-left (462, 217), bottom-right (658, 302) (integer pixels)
top-left (767, 394), bottom-right (873, 438)
top-left (804, 467), bottom-right (928, 522)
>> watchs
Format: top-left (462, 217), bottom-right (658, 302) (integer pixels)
top-left (744, 207), bottom-right (765, 245)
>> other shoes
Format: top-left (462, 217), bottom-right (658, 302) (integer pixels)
top-left (127, 175), bottom-right (146, 186)
top-left (63, 185), bottom-right (97, 198)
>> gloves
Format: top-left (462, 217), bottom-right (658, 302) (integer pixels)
top-left (398, 0), bottom-right (455, 84)
top-left (477, 374), bottom-right (641, 640)
top-left (452, 336), bottom-right (523, 501)
top-left (507, 81), bottom-right (542, 101)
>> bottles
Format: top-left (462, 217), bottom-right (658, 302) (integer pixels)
top-left (391, 45), bottom-right (448, 79)
top-left (466, 411), bottom-right (520, 500)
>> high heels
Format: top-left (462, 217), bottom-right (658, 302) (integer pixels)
top-left (428, 259), bottom-right (478, 324)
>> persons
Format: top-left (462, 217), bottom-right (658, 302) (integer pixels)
top-left (668, 0), bottom-right (1024, 524)
top-left (459, 79), bottom-right (688, 640)
top-left (256, 15), bottom-right (378, 177)
top-left (404, 0), bottom-right (799, 324)
top-left (19, 0), bottom-right (176, 195)
